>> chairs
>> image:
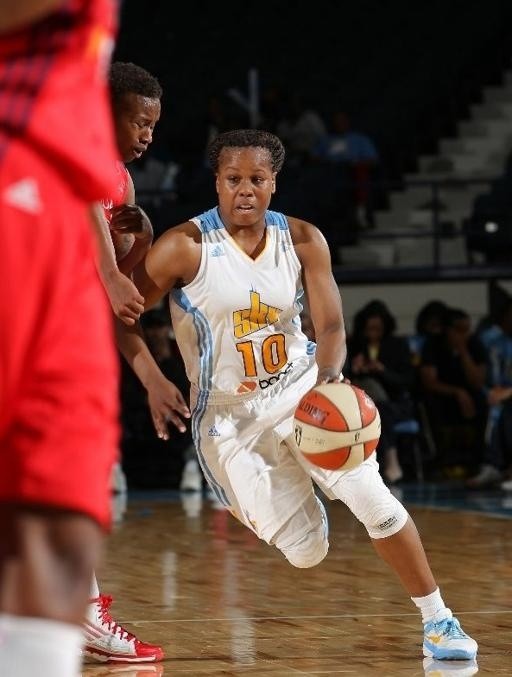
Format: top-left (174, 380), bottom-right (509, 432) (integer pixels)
top-left (362, 324), bottom-right (512, 494)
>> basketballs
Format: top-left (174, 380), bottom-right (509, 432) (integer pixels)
top-left (293, 383), bottom-right (383, 471)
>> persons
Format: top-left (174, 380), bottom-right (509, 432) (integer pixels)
top-left (106, 59), bottom-right (154, 272)
top-left (114, 127), bottom-right (480, 659)
top-left (2, 0), bottom-right (168, 666)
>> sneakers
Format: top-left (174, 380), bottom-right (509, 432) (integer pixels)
top-left (73, 598), bottom-right (166, 667)
top-left (419, 613), bottom-right (479, 663)
top-left (112, 459), bottom-right (204, 494)
